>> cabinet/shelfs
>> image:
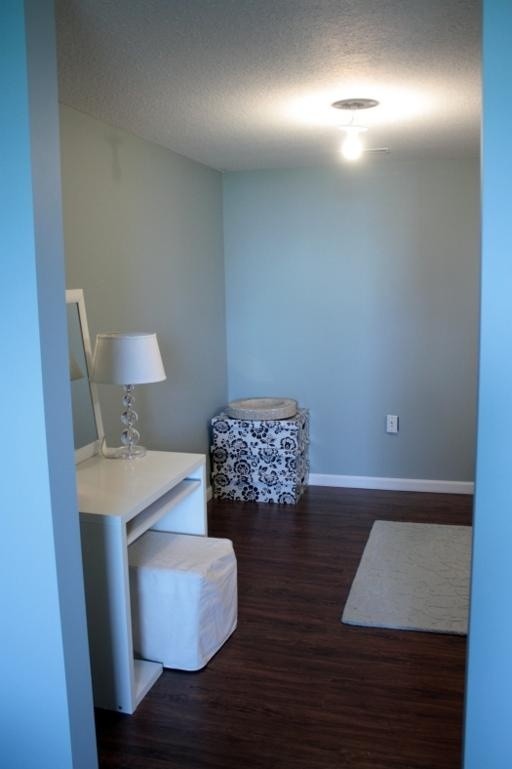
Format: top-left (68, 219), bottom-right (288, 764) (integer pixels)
top-left (76, 449), bottom-right (211, 713)
top-left (211, 406), bottom-right (311, 506)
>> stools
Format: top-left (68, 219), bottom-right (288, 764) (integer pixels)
top-left (127, 530), bottom-right (240, 672)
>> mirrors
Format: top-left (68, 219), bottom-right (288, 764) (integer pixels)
top-left (62, 287), bottom-right (107, 467)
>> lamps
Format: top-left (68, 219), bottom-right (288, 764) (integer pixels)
top-left (87, 330), bottom-right (167, 459)
top-left (330, 98), bottom-right (379, 161)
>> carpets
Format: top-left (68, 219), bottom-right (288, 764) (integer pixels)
top-left (340, 518), bottom-right (472, 636)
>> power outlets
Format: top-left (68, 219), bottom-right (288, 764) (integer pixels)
top-left (387, 414), bottom-right (399, 433)
